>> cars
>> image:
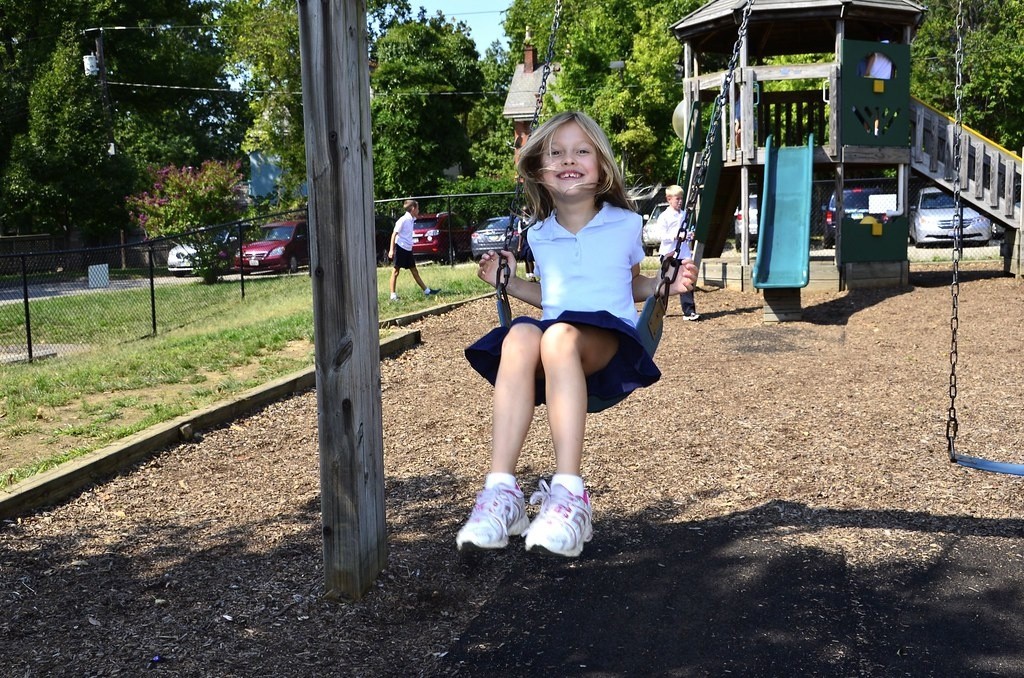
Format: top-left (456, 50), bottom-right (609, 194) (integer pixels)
top-left (471, 217), bottom-right (535, 264)
top-left (907, 186), bottom-right (993, 249)
top-left (639, 202), bottom-right (690, 256)
top-left (373, 211), bottom-right (400, 268)
top-left (167, 230), bottom-right (248, 276)
top-left (234, 220), bottom-right (315, 276)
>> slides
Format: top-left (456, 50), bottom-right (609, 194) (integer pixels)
top-left (752, 132), bottom-right (814, 289)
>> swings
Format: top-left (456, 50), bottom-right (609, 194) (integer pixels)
top-left (496, 0), bottom-right (755, 416)
top-left (946, 2), bottom-right (1024, 476)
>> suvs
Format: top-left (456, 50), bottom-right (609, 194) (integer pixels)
top-left (823, 182), bottom-right (891, 250)
top-left (404, 210), bottom-right (477, 268)
top-left (734, 195), bottom-right (764, 253)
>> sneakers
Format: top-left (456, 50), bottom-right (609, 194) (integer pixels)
top-left (455, 481), bottom-right (527, 549)
top-left (425, 287), bottom-right (441, 297)
top-left (683, 312), bottom-right (702, 321)
top-left (525, 482), bottom-right (595, 559)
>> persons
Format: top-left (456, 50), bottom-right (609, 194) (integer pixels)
top-left (456, 111), bottom-right (698, 562)
top-left (517, 205), bottom-right (539, 283)
top-left (388, 199), bottom-right (442, 302)
top-left (653, 185), bottom-right (704, 321)
